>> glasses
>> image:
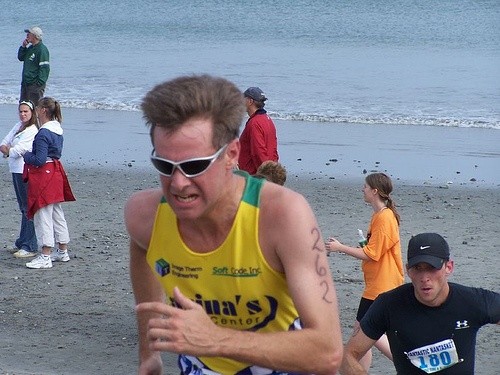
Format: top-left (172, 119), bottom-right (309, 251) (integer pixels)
top-left (150, 143), bottom-right (228, 178)
top-left (19, 99), bottom-right (34, 106)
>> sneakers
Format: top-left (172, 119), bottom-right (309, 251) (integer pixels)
top-left (14, 248), bottom-right (36, 257)
top-left (49, 248), bottom-right (70, 262)
top-left (6, 244), bottom-right (20, 252)
top-left (26, 254), bottom-right (52, 269)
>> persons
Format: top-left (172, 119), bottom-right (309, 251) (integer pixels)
top-left (0, 98), bottom-right (38, 258)
top-left (18, 27), bottom-right (50, 106)
top-left (21, 97), bottom-right (76, 268)
top-left (252, 160), bottom-right (286, 186)
top-left (325, 172), bottom-right (404, 373)
top-left (339, 233), bottom-right (500, 375)
top-left (123, 74), bottom-right (343, 375)
top-left (238, 86), bottom-right (279, 177)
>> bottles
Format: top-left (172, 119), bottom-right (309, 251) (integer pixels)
top-left (357, 229), bottom-right (367, 248)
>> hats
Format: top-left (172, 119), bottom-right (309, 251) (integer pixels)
top-left (407, 233), bottom-right (450, 269)
top-left (24, 27), bottom-right (42, 38)
top-left (241, 87), bottom-right (266, 101)
